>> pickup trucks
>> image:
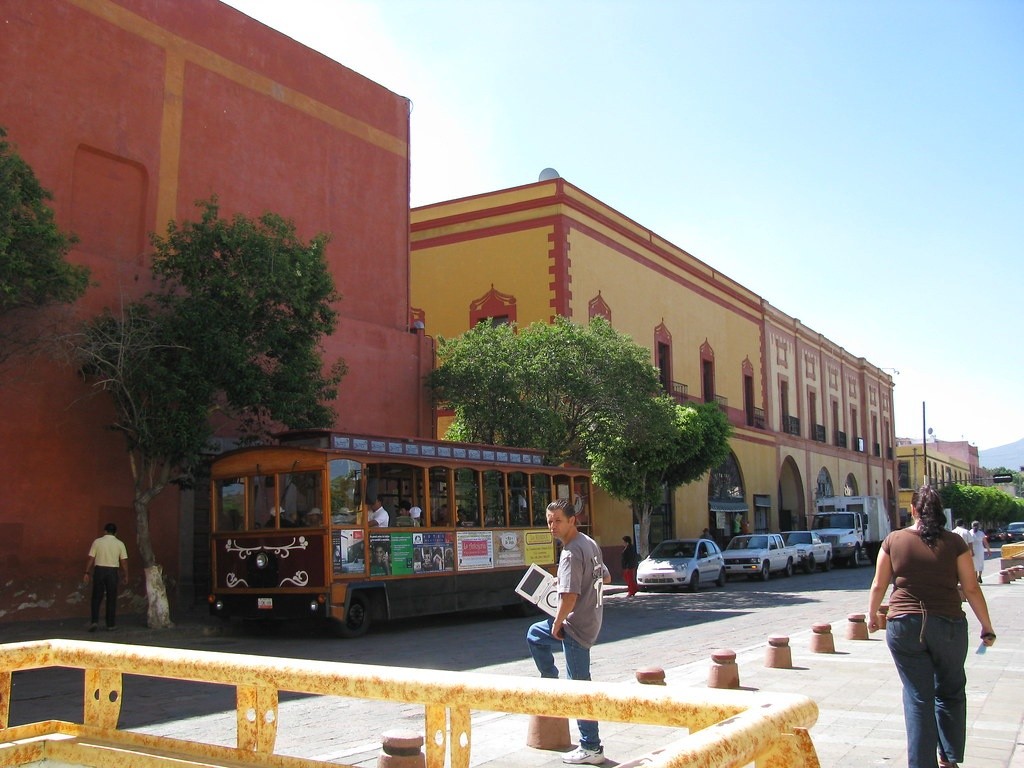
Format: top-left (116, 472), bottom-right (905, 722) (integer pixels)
top-left (778, 531), bottom-right (833, 575)
top-left (720, 533), bottom-right (799, 582)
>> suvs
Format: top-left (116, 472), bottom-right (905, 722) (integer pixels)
top-left (1006, 522), bottom-right (1024, 543)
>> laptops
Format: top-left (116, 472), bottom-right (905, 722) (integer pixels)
top-left (514, 563), bottom-right (559, 617)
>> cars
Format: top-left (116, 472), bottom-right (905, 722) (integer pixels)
top-left (636, 538), bottom-right (727, 593)
top-left (985, 528), bottom-right (1006, 543)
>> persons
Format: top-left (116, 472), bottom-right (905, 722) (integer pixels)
top-left (969, 521), bottom-right (992, 582)
top-left (83, 523), bottom-right (129, 632)
top-left (699, 528), bottom-right (712, 540)
top-left (621, 536), bottom-right (639, 599)
top-left (526, 500), bottom-right (611, 764)
top-left (227, 506), bottom-right (323, 529)
top-left (414, 547), bottom-right (454, 572)
top-left (951, 519), bottom-right (975, 557)
top-left (867, 485), bottom-right (996, 768)
top-left (370, 544), bottom-right (392, 576)
top-left (396, 486), bottom-right (546, 527)
top-left (368, 495), bottom-right (389, 528)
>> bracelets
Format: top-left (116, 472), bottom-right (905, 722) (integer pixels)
top-left (84, 572), bottom-right (89, 574)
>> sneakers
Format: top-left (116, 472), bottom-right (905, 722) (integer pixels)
top-left (563, 746), bottom-right (604, 764)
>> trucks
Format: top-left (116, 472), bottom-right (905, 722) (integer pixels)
top-left (810, 495), bottom-right (893, 569)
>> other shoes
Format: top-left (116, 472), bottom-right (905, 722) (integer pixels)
top-left (628, 593), bottom-right (634, 597)
top-left (978, 577), bottom-right (982, 583)
top-left (106, 626), bottom-right (117, 631)
top-left (89, 623), bottom-right (98, 632)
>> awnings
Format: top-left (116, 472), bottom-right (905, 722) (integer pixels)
top-left (709, 501), bottom-right (749, 513)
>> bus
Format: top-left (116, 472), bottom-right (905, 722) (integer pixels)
top-left (202, 426), bottom-right (599, 640)
